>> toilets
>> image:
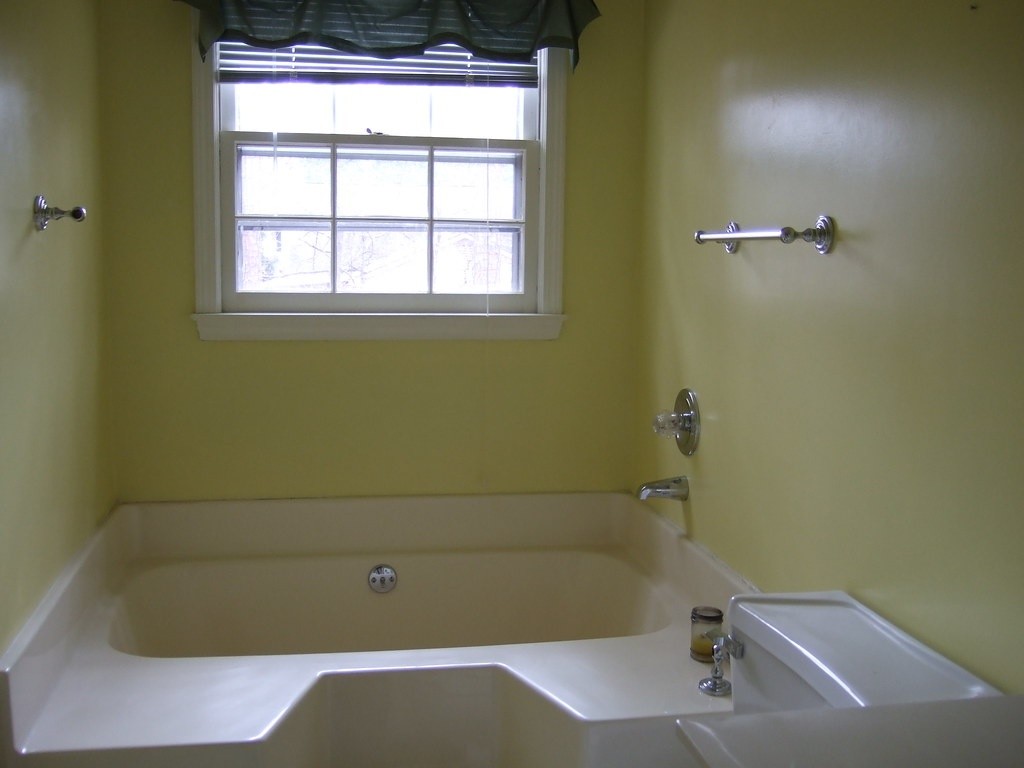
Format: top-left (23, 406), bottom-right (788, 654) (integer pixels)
top-left (731, 590), bottom-right (1006, 713)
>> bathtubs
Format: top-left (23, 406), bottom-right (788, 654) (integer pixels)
top-left (0, 494), bottom-right (766, 764)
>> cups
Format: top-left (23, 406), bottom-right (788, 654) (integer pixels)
top-left (690, 606), bottom-right (723, 661)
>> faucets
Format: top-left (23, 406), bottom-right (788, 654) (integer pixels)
top-left (634, 475), bottom-right (689, 501)
